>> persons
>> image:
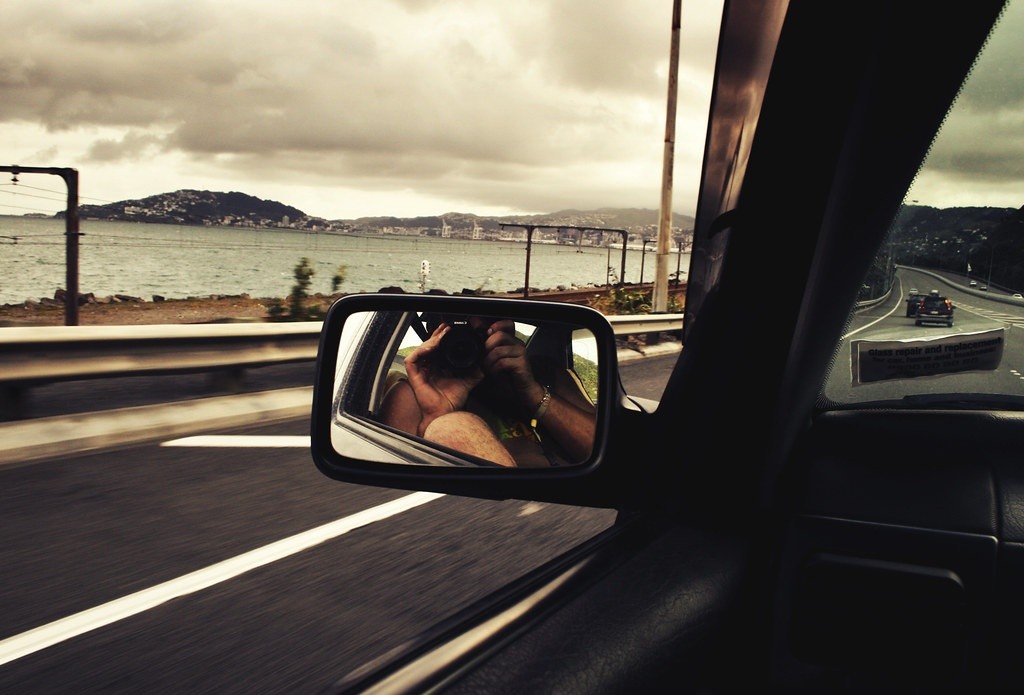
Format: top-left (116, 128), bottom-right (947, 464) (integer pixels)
top-left (376, 313), bottom-right (598, 465)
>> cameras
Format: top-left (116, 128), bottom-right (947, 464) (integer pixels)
top-left (435, 320), bottom-right (499, 371)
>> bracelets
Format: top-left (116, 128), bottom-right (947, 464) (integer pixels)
top-left (531, 385), bottom-right (551, 421)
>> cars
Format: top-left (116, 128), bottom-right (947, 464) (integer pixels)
top-left (969, 280), bottom-right (977, 286)
top-left (1011, 294), bottom-right (1024, 301)
top-left (915, 296), bottom-right (956, 327)
top-left (908, 288), bottom-right (919, 295)
top-left (905, 294), bottom-right (929, 317)
top-left (979, 284), bottom-right (987, 291)
top-left (328, 310), bottom-right (599, 467)
top-left (929, 289), bottom-right (940, 297)
